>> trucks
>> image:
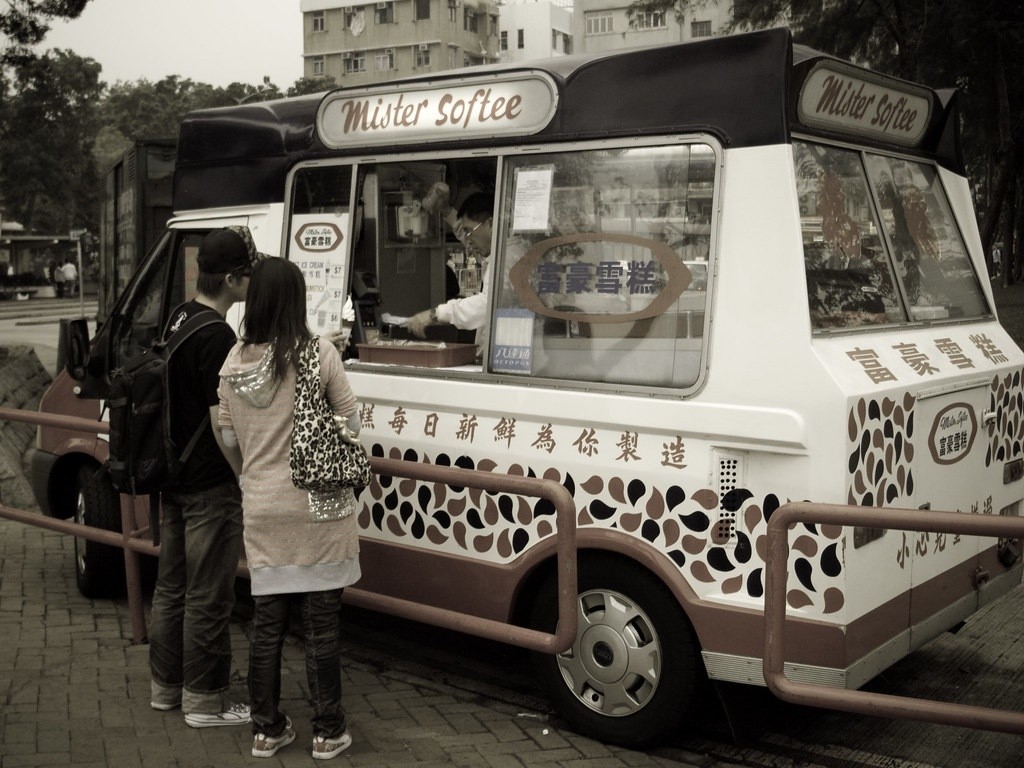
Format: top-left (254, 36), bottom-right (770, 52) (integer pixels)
top-left (22, 26), bottom-right (1024, 750)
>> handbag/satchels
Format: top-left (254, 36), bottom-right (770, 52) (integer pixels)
top-left (290, 335), bottom-right (372, 491)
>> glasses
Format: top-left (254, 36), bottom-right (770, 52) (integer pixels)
top-left (465, 215), bottom-right (490, 240)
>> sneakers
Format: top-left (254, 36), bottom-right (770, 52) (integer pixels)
top-left (312, 728), bottom-right (352, 759)
top-left (252, 715), bottom-right (296, 757)
top-left (151, 702), bottom-right (182, 710)
top-left (185, 701), bottom-right (254, 727)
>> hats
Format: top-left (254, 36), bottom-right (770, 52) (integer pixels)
top-left (197, 225), bottom-right (271, 272)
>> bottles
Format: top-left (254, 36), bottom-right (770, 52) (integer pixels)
top-left (422, 182), bottom-right (449, 210)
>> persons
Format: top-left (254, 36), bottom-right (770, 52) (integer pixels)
top-left (148, 230), bottom-right (271, 729)
top-left (217, 257), bottom-right (361, 762)
top-left (991, 245), bottom-right (1001, 281)
top-left (44, 259), bottom-right (78, 299)
top-left (401, 184), bottom-right (545, 367)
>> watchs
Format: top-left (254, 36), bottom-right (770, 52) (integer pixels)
top-left (430, 307), bottom-right (438, 323)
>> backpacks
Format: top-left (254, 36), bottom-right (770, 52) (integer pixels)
top-left (99, 301), bottom-right (226, 496)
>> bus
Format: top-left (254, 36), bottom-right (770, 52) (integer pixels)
top-left (0, 222), bottom-right (101, 301)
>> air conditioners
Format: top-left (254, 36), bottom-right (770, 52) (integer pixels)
top-left (377, 2), bottom-right (389, 9)
top-left (385, 49), bottom-right (393, 55)
top-left (449, 0), bottom-right (460, 8)
top-left (341, 53), bottom-right (351, 60)
top-left (466, 8), bottom-right (474, 17)
top-left (418, 45), bottom-right (429, 52)
top-left (345, 6), bottom-right (354, 13)
